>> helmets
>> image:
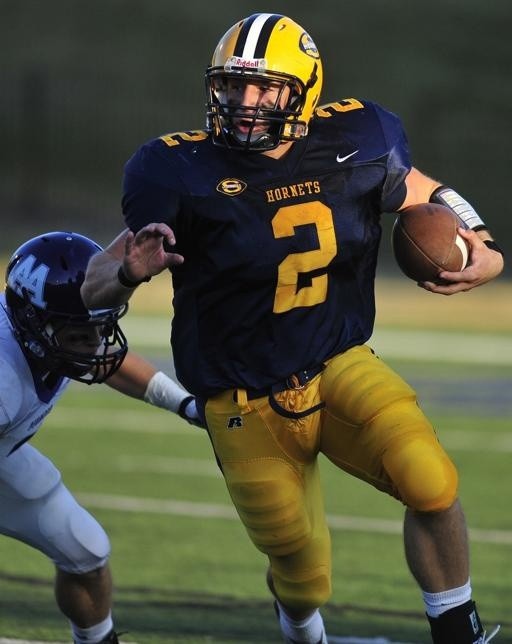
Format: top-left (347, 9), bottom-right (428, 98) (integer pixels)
top-left (6, 231), bottom-right (128, 385)
top-left (203, 13), bottom-right (323, 152)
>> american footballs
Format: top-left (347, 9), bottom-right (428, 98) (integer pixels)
top-left (391, 203), bottom-right (470, 286)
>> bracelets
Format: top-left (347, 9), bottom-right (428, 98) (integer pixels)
top-left (468, 238), bottom-right (504, 263)
top-left (116, 267), bottom-right (156, 289)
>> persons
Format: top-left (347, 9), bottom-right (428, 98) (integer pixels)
top-left (80, 9), bottom-right (507, 643)
top-left (1, 231), bottom-right (207, 644)
